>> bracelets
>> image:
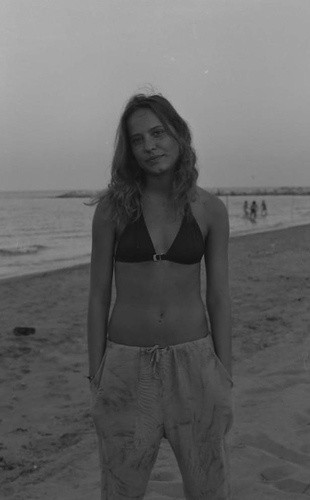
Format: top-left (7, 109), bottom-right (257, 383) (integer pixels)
top-left (85, 375), bottom-right (94, 380)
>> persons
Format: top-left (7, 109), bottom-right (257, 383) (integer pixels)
top-left (84, 91), bottom-right (236, 500)
